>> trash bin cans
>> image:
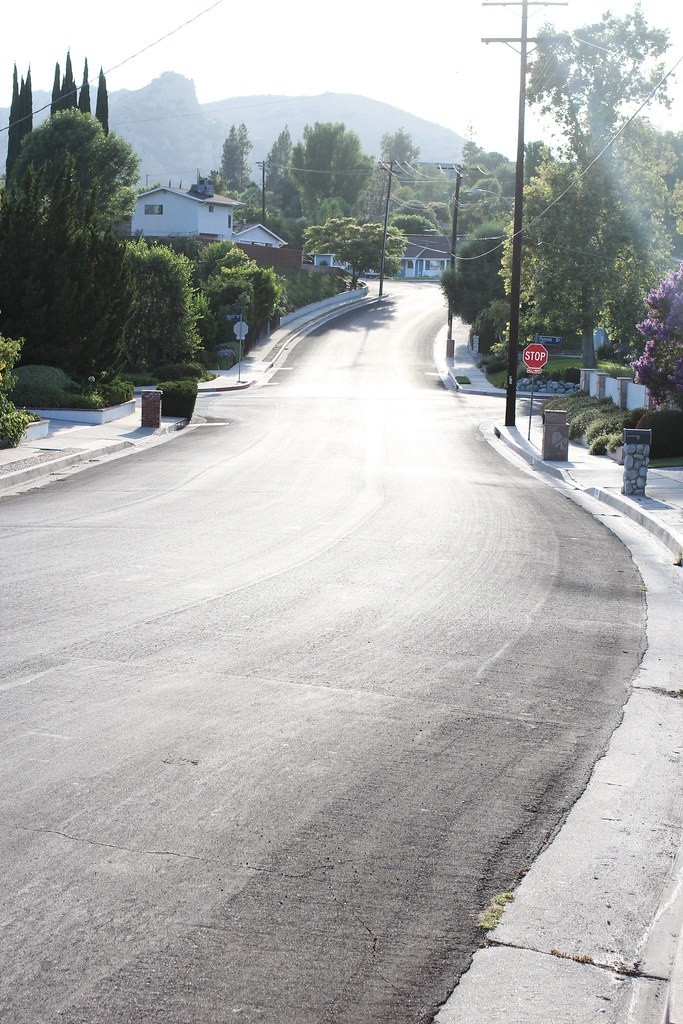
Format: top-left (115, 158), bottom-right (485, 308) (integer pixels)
top-left (538, 408), bottom-right (572, 462)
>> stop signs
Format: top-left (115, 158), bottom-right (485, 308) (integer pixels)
top-left (522, 344), bottom-right (548, 368)
